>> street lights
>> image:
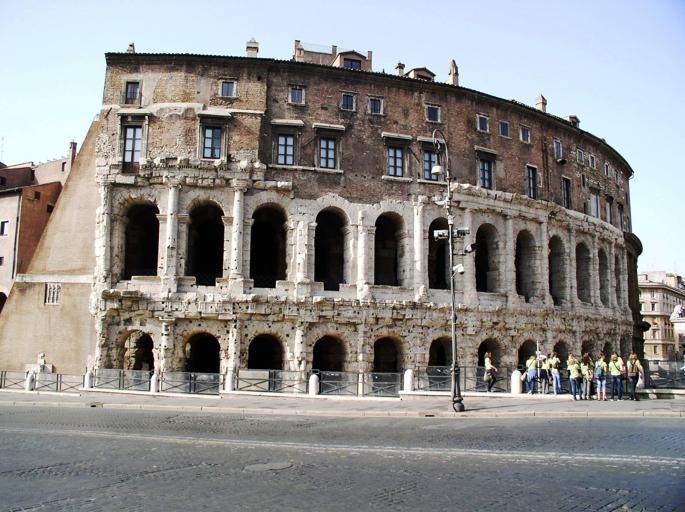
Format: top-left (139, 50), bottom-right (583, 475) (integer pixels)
top-left (430, 127), bottom-right (469, 412)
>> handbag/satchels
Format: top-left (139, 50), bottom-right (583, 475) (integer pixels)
top-left (636, 379), bottom-right (645, 388)
top-left (620, 370), bottom-right (627, 378)
top-left (585, 369), bottom-right (594, 381)
top-left (553, 362), bottom-right (563, 369)
top-left (483, 373), bottom-right (493, 381)
top-left (520, 371), bottom-right (527, 382)
top-left (628, 371), bottom-right (637, 377)
top-left (575, 377), bottom-right (582, 388)
top-left (547, 375), bottom-right (553, 382)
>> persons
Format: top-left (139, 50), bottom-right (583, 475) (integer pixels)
top-left (566, 350), bottom-right (644, 401)
top-left (524, 355), bottom-right (538, 395)
top-left (548, 351), bottom-right (563, 395)
top-left (540, 357), bottom-right (550, 393)
top-left (484, 351), bottom-right (498, 392)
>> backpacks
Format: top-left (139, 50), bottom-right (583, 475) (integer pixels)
top-left (594, 365), bottom-right (605, 380)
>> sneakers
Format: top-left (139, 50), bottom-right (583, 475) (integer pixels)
top-left (528, 390), bottom-right (563, 395)
top-left (572, 396), bottom-right (640, 401)
top-left (486, 390), bottom-right (493, 392)
